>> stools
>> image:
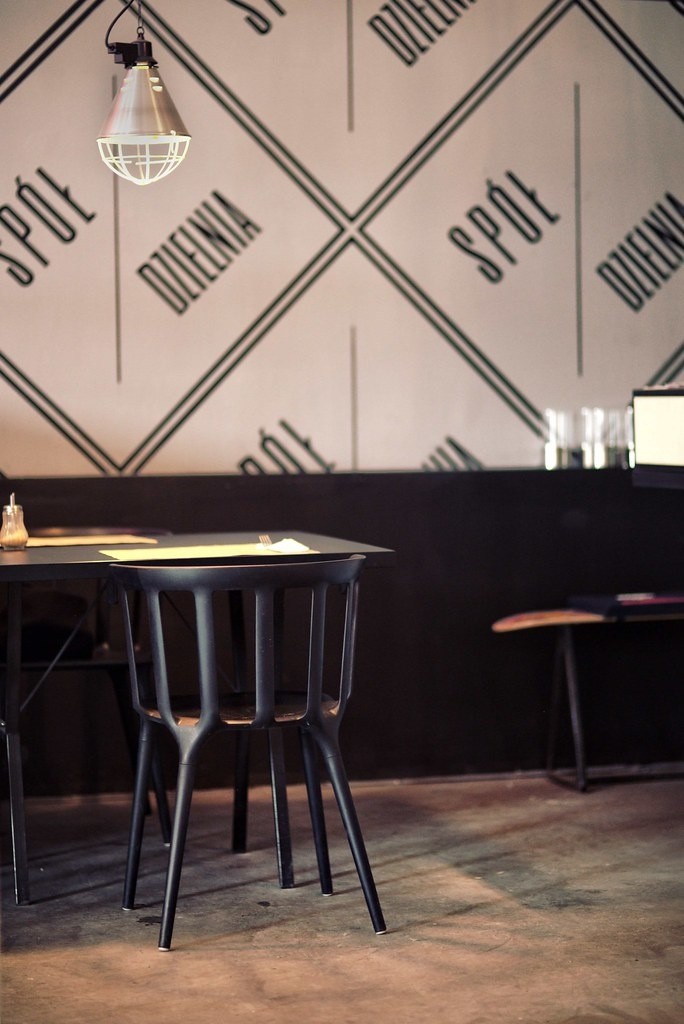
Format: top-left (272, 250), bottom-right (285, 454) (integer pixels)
top-left (491, 605), bottom-right (684, 793)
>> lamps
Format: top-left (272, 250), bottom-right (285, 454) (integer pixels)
top-left (97, 0), bottom-right (192, 186)
top-left (630, 388), bottom-right (684, 485)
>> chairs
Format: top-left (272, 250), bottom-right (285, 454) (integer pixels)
top-left (22, 528), bottom-right (387, 952)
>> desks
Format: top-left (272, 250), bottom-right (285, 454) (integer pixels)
top-left (0, 531), bottom-right (399, 904)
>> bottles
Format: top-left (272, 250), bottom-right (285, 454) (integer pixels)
top-left (0, 492), bottom-right (28, 550)
